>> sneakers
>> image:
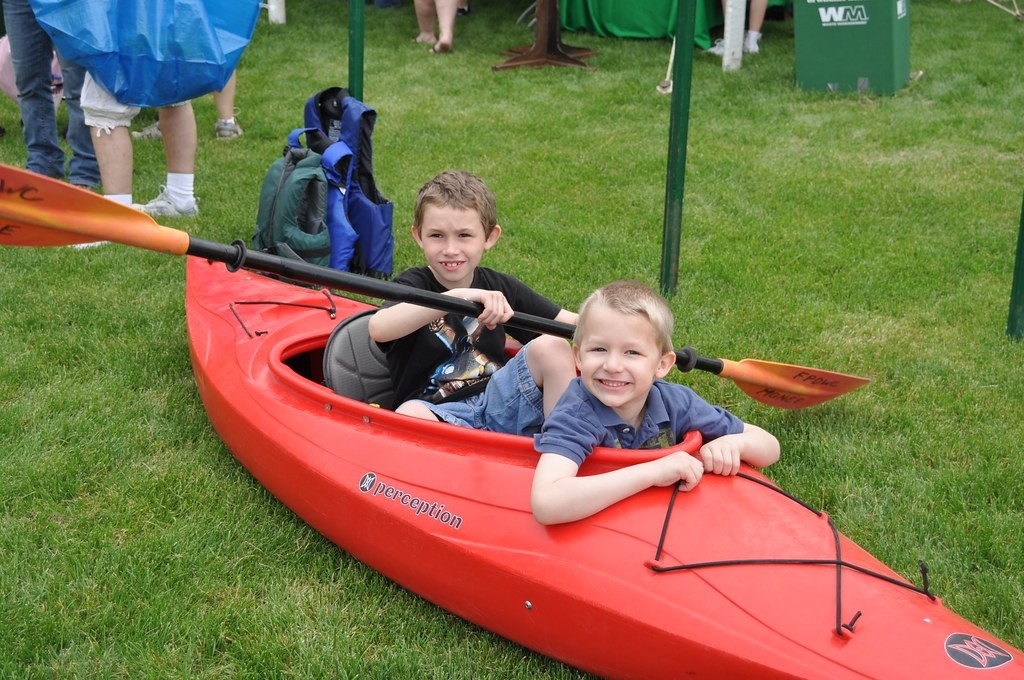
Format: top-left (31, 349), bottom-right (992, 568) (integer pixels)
top-left (215, 116), bottom-right (243, 140)
top-left (708, 38), bottom-right (724, 55)
top-left (743, 35), bottom-right (762, 53)
top-left (142, 185), bottom-right (200, 217)
top-left (68, 240), bottom-right (113, 250)
top-left (131, 121), bottom-right (162, 139)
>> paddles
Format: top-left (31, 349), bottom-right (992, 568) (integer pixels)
top-left (1, 163), bottom-right (871, 410)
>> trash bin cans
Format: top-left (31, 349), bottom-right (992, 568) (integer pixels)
top-left (793, 0), bottom-right (912, 96)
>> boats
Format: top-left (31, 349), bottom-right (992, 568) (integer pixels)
top-left (183, 252), bottom-right (1024, 680)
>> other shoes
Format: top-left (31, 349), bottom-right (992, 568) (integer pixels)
top-left (412, 37), bottom-right (454, 54)
top-left (457, 4), bottom-right (470, 16)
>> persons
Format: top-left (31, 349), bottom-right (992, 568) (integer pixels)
top-left (367, 170), bottom-right (580, 438)
top-left (531, 280), bottom-right (781, 525)
top-left (0, 0), bottom-right (768, 251)
